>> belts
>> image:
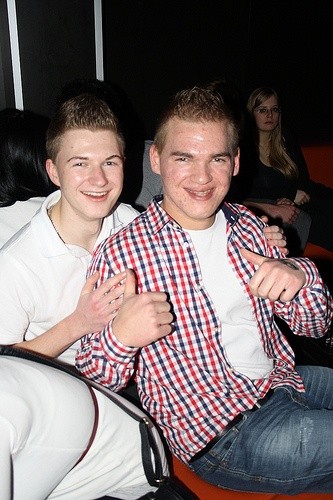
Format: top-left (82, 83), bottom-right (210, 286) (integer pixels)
top-left (188, 389), bottom-right (274, 463)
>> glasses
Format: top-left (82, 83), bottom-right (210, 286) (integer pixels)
top-left (251, 107), bottom-right (279, 113)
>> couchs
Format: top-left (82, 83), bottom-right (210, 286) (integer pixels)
top-left (0, 107), bottom-right (60, 208)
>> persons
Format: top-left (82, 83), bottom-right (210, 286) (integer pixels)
top-left (75, 86), bottom-right (333, 493)
top-left (0, 96), bottom-right (142, 406)
top-left (230, 87), bottom-right (311, 257)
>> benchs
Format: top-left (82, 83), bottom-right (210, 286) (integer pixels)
top-left (296, 145), bottom-right (333, 259)
